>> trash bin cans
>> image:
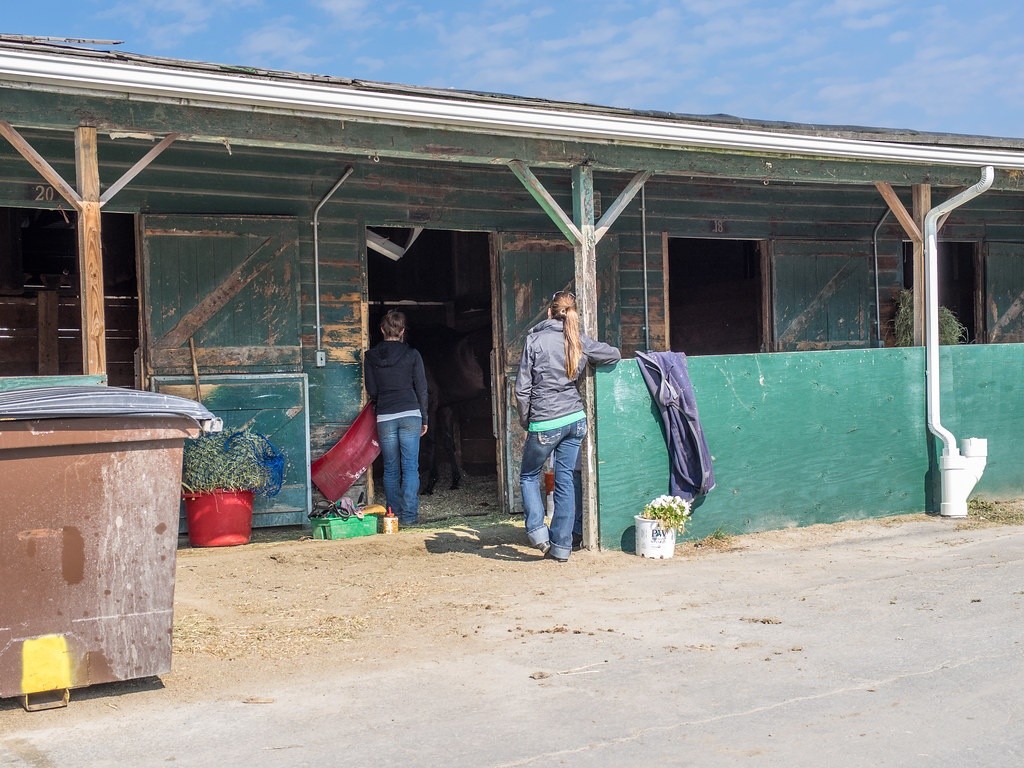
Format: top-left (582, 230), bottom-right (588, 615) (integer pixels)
top-left (0, 384), bottom-right (216, 700)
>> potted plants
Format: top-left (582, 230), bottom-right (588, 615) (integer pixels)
top-left (176, 418), bottom-right (273, 547)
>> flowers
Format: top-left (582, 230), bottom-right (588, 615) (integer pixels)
top-left (642, 494), bottom-right (694, 535)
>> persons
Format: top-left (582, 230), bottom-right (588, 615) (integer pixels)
top-left (514, 291), bottom-right (621, 561)
top-left (364, 311), bottom-right (428, 526)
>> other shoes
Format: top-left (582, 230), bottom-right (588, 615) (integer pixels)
top-left (543, 552), bottom-right (568, 562)
top-left (536, 541), bottom-right (551, 553)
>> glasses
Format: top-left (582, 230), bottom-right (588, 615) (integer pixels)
top-left (552, 290), bottom-right (576, 302)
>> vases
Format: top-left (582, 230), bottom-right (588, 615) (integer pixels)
top-left (633, 515), bottom-right (676, 559)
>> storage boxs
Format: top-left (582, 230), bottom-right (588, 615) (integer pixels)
top-left (311, 514), bottom-right (379, 540)
